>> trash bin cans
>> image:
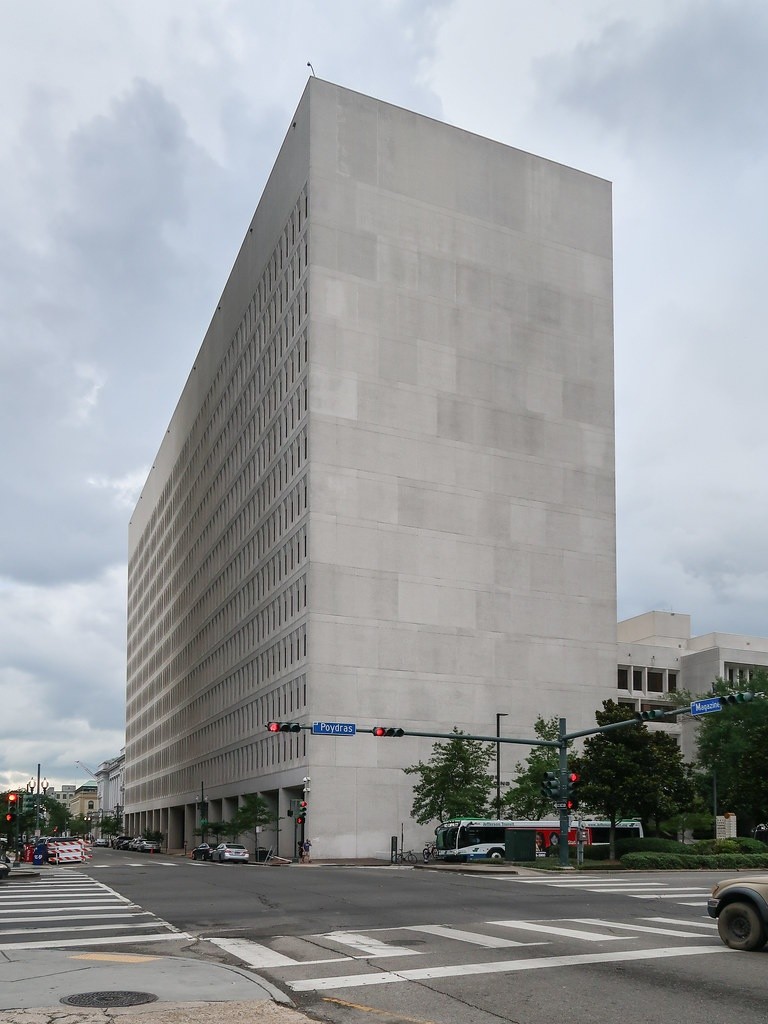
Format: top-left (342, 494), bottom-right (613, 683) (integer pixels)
top-left (255, 847), bottom-right (266, 862)
top-left (23, 842), bottom-right (34, 862)
top-left (33, 847), bottom-right (43, 865)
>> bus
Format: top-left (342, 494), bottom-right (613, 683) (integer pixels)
top-left (434, 816), bottom-right (645, 862)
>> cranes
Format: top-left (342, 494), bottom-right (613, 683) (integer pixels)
top-left (74, 759), bottom-right (97, 781)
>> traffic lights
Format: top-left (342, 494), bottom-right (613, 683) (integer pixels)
top-left (267, 722), bottom-right (301, 733)
top-left (297, 817), bottom-right (305, 824)
top-left (7, 793), bottom-right (18, 815)
top-left (565, 771), bottom-right (579, 810)
top-left (718, 692), bottom-right (754, 707)
top-left (633, 709), bottom-right (664, 722)
top-left (372, 726), bottom-right (405, 738)
top-left (298, 800), bottom-right (307, 817)
top-left (5, 813), bottom-right (16, 822)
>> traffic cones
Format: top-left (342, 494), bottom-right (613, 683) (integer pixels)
top-left (150, 847), bottom-right (154, 855)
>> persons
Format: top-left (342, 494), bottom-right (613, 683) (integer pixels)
top-left (298, 839), bottom-right (312, 864)
top-left (536, 832), bottom-right (547, 852)
top-left (549, 832), bottom-right (560, 845)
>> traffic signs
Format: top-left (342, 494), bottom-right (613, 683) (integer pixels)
top-left (552, 801), bottom-right (567, 810)
top-left (540, 771), bottom-right (559, 798)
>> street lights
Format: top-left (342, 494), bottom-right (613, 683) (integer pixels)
top-left (496, 712), bottom-right (510, 820)
top-left (83, 812), bottom-right (91, 834)
top-left (195, 780), bottom-right (208, 844)
top-left (27, 763), bottom-right (49, 846)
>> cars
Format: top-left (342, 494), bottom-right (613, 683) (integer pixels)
top-left (112, 836), bottom-right (161, 853)
top-left (0, 835), bottom-right (84, 863)
top-left (210, 842), bottom-right (250, 864)
top-left (707, 874), bottom-right (768, 953)
top-left (93, 839), bottom-right (109, 848)
top-left (191, 842), bottom-right (219, 861)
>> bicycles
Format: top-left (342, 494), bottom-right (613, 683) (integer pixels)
top-left (422, 841), bottom-right (437, 860)
top-left (392, 849), bottom-right (418, 864)
